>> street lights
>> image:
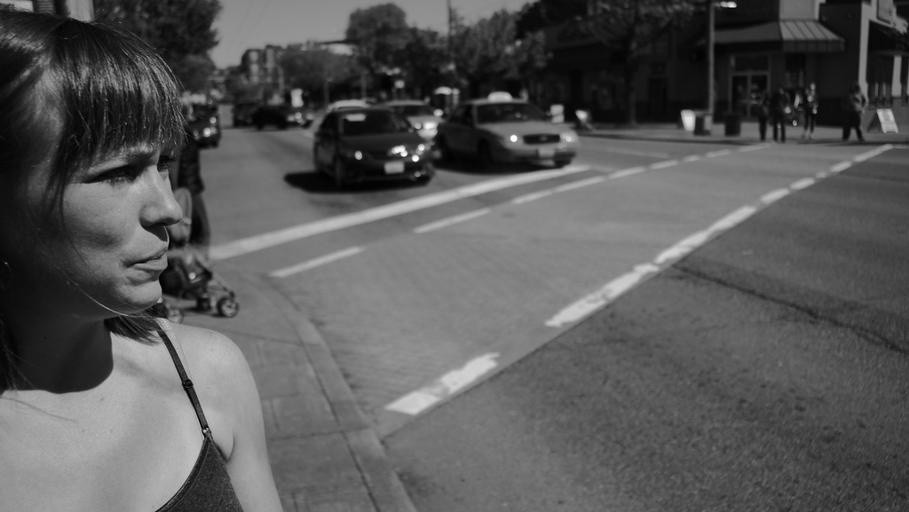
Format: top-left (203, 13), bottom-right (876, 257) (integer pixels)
top-left (702, 1), bottom-right (738, 135)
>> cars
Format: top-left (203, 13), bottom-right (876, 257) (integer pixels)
top-left (381, 99), bottom-right (445, 151)
top-left (433, 99), bottom-right (577, 172)
top-left (312, 107), bottom-right (429, 189)
top-left (182, 102), bottom-right (220, 149)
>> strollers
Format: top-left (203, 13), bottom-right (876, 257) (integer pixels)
top-left (159, 187), bottom-right (239, 324)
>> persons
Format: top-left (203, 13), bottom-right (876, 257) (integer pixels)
top-left (840, 81), bottom-right (867, 144)
top-left (1, 6), bottom-right (287, 512)
top-left (168, 114), bottom-right (214, 268)
top-left (758, 96), bottom-right (771, 141)
top-left (545, 99), bottom-right (566, 125)
top-left (768, 86), bottom-right (789, 143)
top-left (799, 80), bottom-right (819, 141)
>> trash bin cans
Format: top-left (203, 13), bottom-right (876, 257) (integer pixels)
top-left (694, 112), bottom-right (741, 136)
